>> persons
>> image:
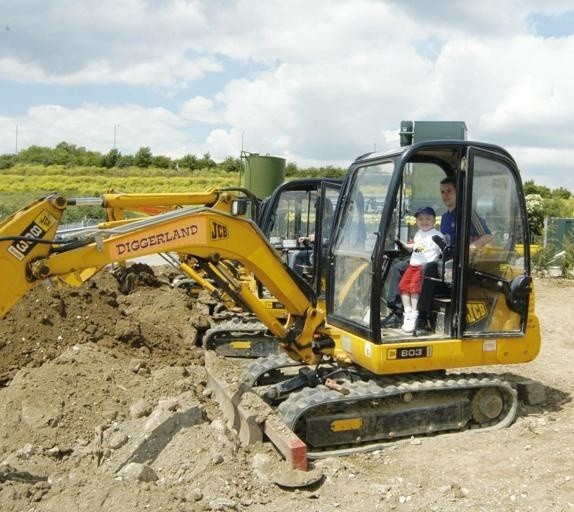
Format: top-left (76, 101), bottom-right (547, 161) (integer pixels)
top-left (285, 197), bottom-right (337, 274)
top-left (380, 176), bottom-right (494, 324)
top-left (389, 206), bottom-right (448, 336)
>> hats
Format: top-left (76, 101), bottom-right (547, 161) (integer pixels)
top-left (414, 206), bottom-right (435, 216)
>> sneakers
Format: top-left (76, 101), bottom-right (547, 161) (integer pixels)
top-left (402, 308), bottom-right (419, 333)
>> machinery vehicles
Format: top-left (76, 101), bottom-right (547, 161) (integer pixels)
top-left (102, 178), bottom-right (370, 359)
top-left (0, 120), bottom-right (542, 487)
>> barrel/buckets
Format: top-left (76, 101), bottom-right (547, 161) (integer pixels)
top-left (240, 153), bottom-right (285, 204)
